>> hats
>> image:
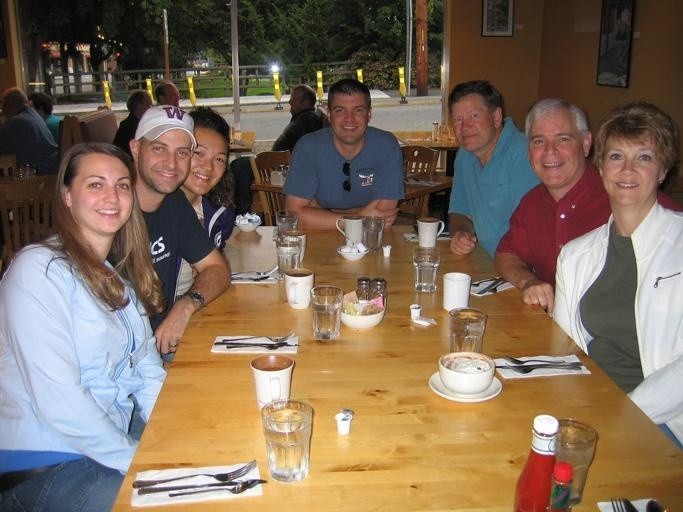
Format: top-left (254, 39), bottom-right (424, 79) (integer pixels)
top-left (134, 105), bottom-right (197, 152)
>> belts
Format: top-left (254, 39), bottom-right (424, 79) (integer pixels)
top-left (0, 465), bottom-right (55, 493)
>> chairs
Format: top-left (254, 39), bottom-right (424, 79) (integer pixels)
top-left (0, 101), bottom-right (456, 277)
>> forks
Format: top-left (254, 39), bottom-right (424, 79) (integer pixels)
top-left (133, 460), bottom-right (259, 488)
top-left (609, 496), bottom-right (625, 512)
top-left (500, 352), bottom-right (565, 364)
top-left (222, 331), bottom-right (297, 342)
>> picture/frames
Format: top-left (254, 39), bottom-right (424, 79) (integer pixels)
top-left (594, 0), bottom-right (634, 88)
top-left (480, 0), bottom-right (514, 37)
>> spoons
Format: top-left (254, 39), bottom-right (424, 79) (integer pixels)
top-left (508, 365), bottom-right (582, 375)
top-left (645, 496), bottom-right (667, 511)
top-left (227, 341), bottom-right (286, 350)
top-left (168, 479), bottom-right (257, 498)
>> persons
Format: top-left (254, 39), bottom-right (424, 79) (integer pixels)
top-left (553, 101), bottom-right (683, 452)
top-left (174, 107), bottom-right (235, 303)
top-left (447, 80), bottom-right (542, 260)
top-left (107, 104), bottom-right (231, 362)
top-left (495, 97), bottom-right (683, 319)
top-left (281, 79), bottom-right (405, 231)
top-left (0, 141), bottom-right (167, 512)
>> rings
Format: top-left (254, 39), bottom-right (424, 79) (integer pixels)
top-left (170, 345), bottom-right (177, 347)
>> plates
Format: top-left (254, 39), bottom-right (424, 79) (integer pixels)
top-left (427, 371), bottom-right (502, 405)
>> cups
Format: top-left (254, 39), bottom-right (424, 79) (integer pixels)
top-left (411, 248), bottom-right (442, 295)
top-left (335, 413), bottom-right (350, 436)
top-left (410, 307), bottom-right (421, 320)
top-left (247, 354), bottom-right (295, 416)
top-left (441, 272), bottom-right (470, 313)
top-left (415, 216), bottom-right (444, 247)
top-left (336, 215), bottom-right (363, 246)
top-left (448, 306), bottom-right (488, 355)
top-left (262, 399), bottom-right (313, 484)
top-left (271, 211), bottom-right (312, 309)
top-left (310, 285), bottom-right (341, 340)
top-left (555, 420), bottom-right (600, 504)
top-left (382, 244), bottom-right (391, 256)
top-left (361, 215), bottom-right (385, 252)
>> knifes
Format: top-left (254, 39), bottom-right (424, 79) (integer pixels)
top-left (495, 360), bottom-right (583, 371)
top-left (136, 479), bottom-right (266, 493)
top-left (477, 277), bottom-right (504, 294)
top-left (213, 342), bottom-right (298, 348)
top-left (623, 498), bottom-right (634, 510)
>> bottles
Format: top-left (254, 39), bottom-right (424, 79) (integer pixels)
top-left (547, 455), bottom-right (572, 512)
top-left (511, 413), bottom-right (572, 511)
top-left (357, 277), bottom-right (372, 298)
top-left (430, 123), bottom-right (455, 143)
top-left (373, 276), bottom-right (386, 308)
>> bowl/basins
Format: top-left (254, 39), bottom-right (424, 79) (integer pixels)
top-left (336, 246), bottom-right (369, 262)
top-left (231, 223), bottom-right (262, 232)
top-left (439, 350), bottom-right (497, 396)
top-left (336, 301), bottom-right (385, 329)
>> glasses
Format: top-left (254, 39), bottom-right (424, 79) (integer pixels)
top-left (342, 162), bottom-right (350, 192)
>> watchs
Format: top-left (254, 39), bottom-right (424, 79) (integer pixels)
top-left (187, 291), bottom-right (205, 309)
